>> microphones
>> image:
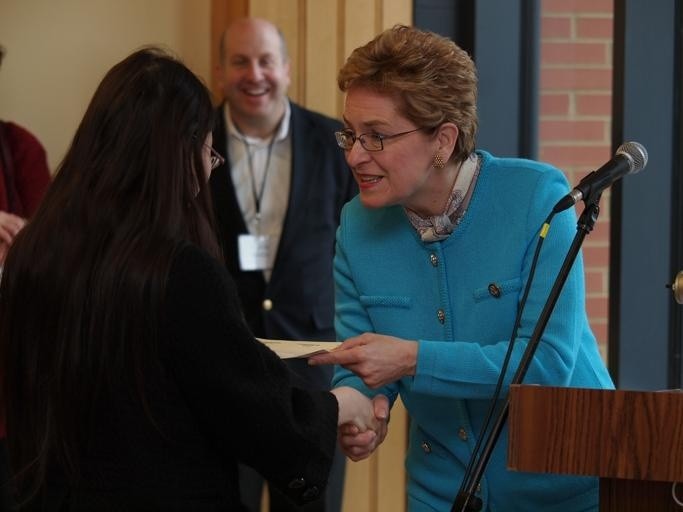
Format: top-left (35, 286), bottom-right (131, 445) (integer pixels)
top-left (557, 139), bottom-right (652, 216)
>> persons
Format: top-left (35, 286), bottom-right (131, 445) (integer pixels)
top-left (198, 17), bottom-right (361, 511)
top-left (0, 118), bottom-right (54, 273)
top-left (1, 44), bottom-right (388, 512)
top-left (326, 24), bottom-right (616, 511)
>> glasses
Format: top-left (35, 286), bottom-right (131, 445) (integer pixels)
top-left (334, 128), bottom-right (419, 152)
top-left (201, 144), bottom-right (224, 170)
